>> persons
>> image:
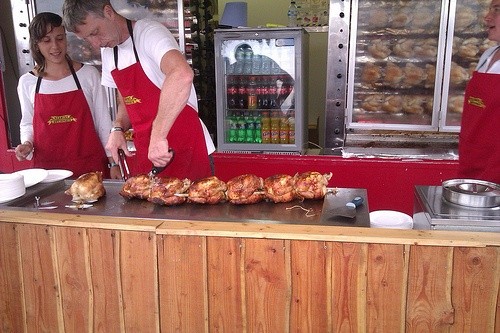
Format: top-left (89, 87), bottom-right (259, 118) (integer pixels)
top-left (63, 0), bottom-right (216, 182)
top-left (14, 12), bottom-right (123, 181)
top-left (223, 44), bottom-right (295, 130)
top-left (456, 0), bottom-right (500, 183)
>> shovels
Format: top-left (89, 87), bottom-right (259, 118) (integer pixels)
top-left (326, 197), bottom-right (363, 218)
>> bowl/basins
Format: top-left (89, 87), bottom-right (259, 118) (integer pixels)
top-left (369, 210), bottom-right (413, 230)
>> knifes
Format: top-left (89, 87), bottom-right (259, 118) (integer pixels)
top-left (147, 147), bottom-right (173, 179)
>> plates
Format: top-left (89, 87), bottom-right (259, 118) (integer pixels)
top-left (0, 173), bottom-right (26, 203)
top-left (12, 168), bottom-right (48, 187)
top-left (40, 169), bottom-right (73, 183)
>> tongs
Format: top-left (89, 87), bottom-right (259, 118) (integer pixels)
top-left (117, 149), bottom-right (131, 182)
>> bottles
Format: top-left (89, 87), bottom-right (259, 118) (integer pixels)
top-left (288, 1), bottom-right (298, 28)
top-left (223, 45), bottom-right (296, 144)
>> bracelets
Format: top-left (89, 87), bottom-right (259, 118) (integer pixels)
top-left (108, 162), bottom-right (119, 168)
top-left (109, 127), bottom-right (124, 133)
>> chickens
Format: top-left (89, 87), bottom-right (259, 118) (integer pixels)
top-left (64, 171), bottom-right (106, 204)
top-left (118, 172), bottom-right (333, 206)
top-left (360, 0), bottom-right (499, 115)
